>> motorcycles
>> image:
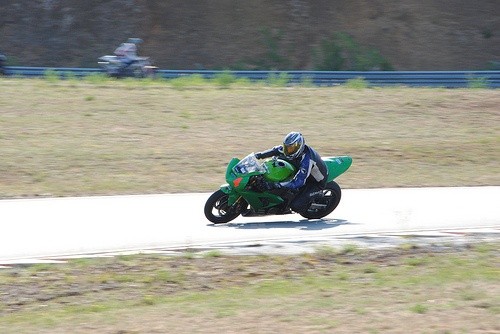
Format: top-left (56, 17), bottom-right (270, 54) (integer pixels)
top-left (97, 54), bottom-right (157, 78)
top-left (203, 155), bottom-right (353, 224)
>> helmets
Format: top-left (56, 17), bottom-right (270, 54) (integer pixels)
top-left (283, 131), bottom-right (305, 162)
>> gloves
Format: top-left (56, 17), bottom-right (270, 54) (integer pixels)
top-left (264, 182), bottom-right (283, 191)
top-left (255, 153), bottom-right (262, 159)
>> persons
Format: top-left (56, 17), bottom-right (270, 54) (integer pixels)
top-left (255, 132), bottom-right (329, 213)
top-left (108, 38), bottom-right (147, 77)
